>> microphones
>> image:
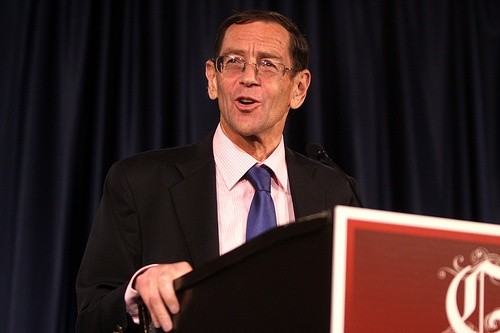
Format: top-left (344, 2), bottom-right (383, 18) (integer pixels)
top-left (306, 142), bottom-right (363, 208)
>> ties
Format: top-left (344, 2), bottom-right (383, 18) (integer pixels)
top-left (246, 166), bottom-right (278, 242)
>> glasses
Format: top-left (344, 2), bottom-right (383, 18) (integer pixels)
top-left (211, 56), bottom-right (294, 82)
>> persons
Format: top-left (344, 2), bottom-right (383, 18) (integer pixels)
top-left (76, 10), bottom-right (364, 333)
